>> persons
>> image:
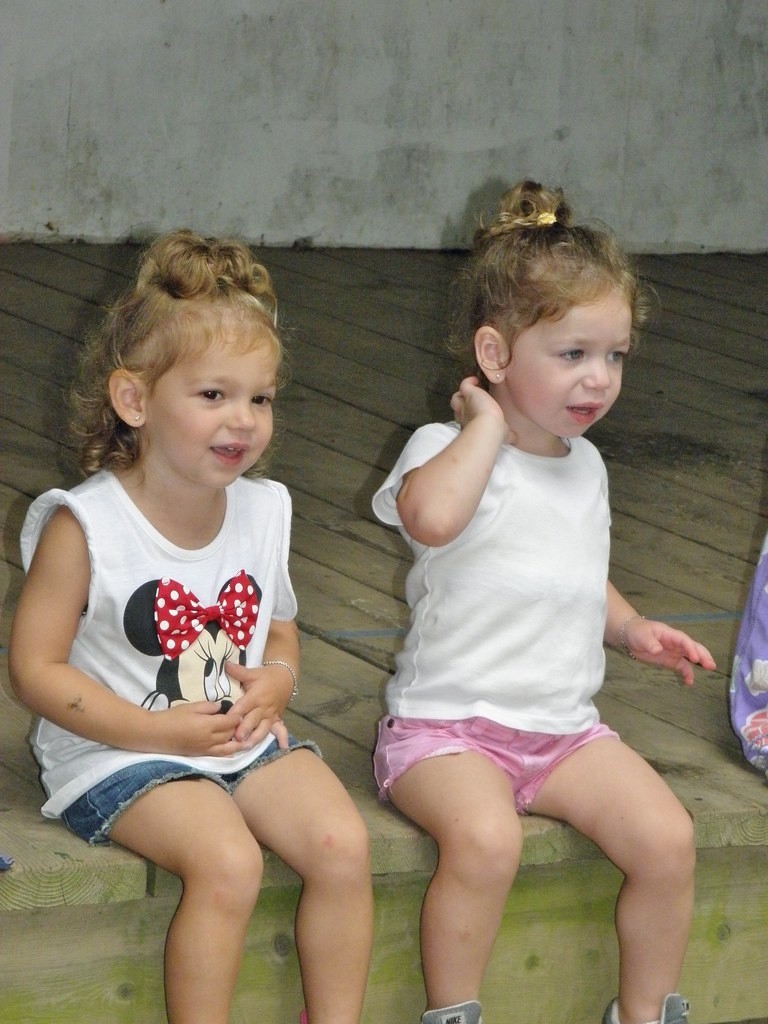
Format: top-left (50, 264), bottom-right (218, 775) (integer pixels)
top-left (6, 230), bottom-right (373, 1024)
top-left (372, 177), bottom-right (700, 1024)
top-left (731, 529), bottom-right (768, 774)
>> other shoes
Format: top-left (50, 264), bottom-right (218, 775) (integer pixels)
top-left (601, 992), bottom-right (689, 1024)
top-left (422, 1000), bottom-right (483, 1024)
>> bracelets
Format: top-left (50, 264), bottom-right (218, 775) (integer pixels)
top-left (621, 613), bottom-right (648, 660)
top-left (267, 659), bottom-right (300, 704)
